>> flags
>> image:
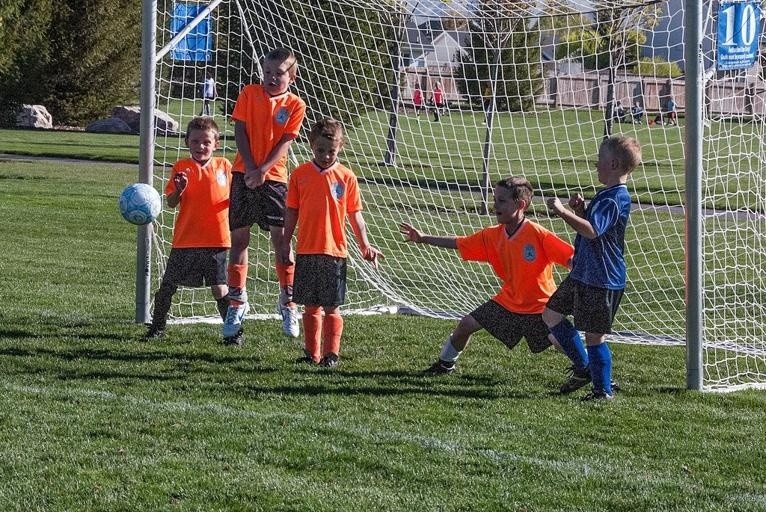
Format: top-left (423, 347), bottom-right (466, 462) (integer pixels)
top-left (169, 3), bottom-right (213, 62)
top-left (716, 0), bottom-right (761, 71)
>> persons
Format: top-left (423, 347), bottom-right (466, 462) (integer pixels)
top-left (197, 71), bottom-right (218, 118)
top-left (218, 46), bottom-right (309, 342)
top-left (412, 82), bottom-right (425, 124)
top-left (396, 174), bottom-right (626, 392)
top-left (138, 115), bottom-right (246, 348)
top-left (277, 116), bottom-right (387, 371)
top-left (429, 81), bottom-right (446, 123)
top-left (614, 94), bottom-right (679, 128)
top-left (541, 134), bottom-right (647, 403)
top-left (480, 83), bottom-right (493, 125)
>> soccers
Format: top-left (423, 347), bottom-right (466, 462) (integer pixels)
top-left (118, 182), bottom-right (161, 226)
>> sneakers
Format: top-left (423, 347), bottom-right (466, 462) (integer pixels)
top-left (141, 321), bottom-right (168, 344)
top-left (296, 347), bottom-right (320, 366)
top-left (558, 370), bottom-right (591, 394)
top-left (424, 359), bottom-right (457, 375)
top-left (280, 304), bottom-right (303, 339)
top-left (585, 389), bottom-right (616, 402)
top-left (321, 352), bottom-right (341, 368)
top-left (220, 303), bottom-right (249, 346)
